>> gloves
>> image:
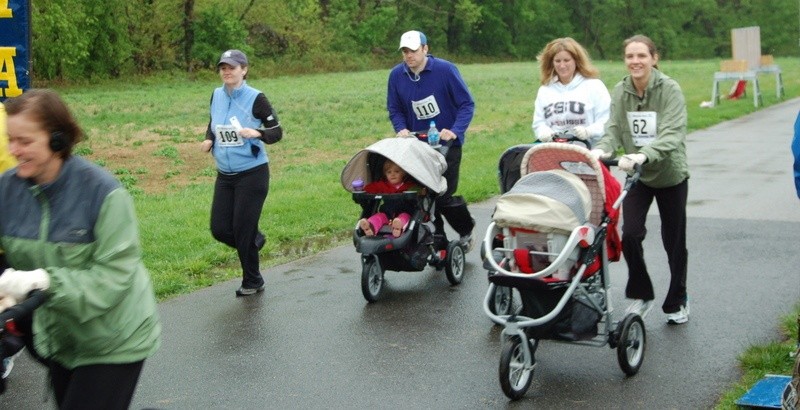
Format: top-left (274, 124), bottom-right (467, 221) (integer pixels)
top-left (573, 126), bottom-right (591, 140)
top-left (0, 268), bottom-right (50, 311)
top-left (535, 124), bottom-right (554, 142)
top-left (618, 153), bottom-right (647, 171)
top-left (579, 148), bottom-right (607, 165)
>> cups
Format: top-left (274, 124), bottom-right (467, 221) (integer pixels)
top-left (351, 178), bottom-right (364, 192)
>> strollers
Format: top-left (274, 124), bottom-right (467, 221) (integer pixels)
top-left (0, 290), bottom-right (50, 394)
top-left (480, 136), bottom-right (648, 402)
top-left (340, 131), bottom-right (467, 304)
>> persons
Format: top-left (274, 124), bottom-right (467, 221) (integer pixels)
top-left (201, 50), bottom-right (283, 296)
top-left (531, 37), bottom-right (612, 174)
top-left (360, 159), bottom-right (426, 238)
top-left (590, 34), bottom-right (690, 324)
top-left (0, 91), bottom-right (161, 410)
top-left (385, 30), bottom-right (475, 260)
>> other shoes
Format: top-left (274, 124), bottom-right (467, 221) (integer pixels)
top-left (625, 299), bottom-right (655, 320)
top-left (256, 235), bottom-right (266, 251)
top-left (235, 285), bottom-right (265, 296)
top-left (460, 216), bottom-right (475, 254)
top-left (392, 217), bottom-right (402, 238)
top-left (359, 219), bottom-right (373, 236)
top-left (665, 301), bottom-right (690, 325)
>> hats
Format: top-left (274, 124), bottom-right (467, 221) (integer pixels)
top-left (216, 50), bottom-right (247, 67)
top-left (398, 30), bottom-right (427, 51)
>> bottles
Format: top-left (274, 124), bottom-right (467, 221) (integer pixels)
top-left (428, 120), bottom-right (440, 147)
